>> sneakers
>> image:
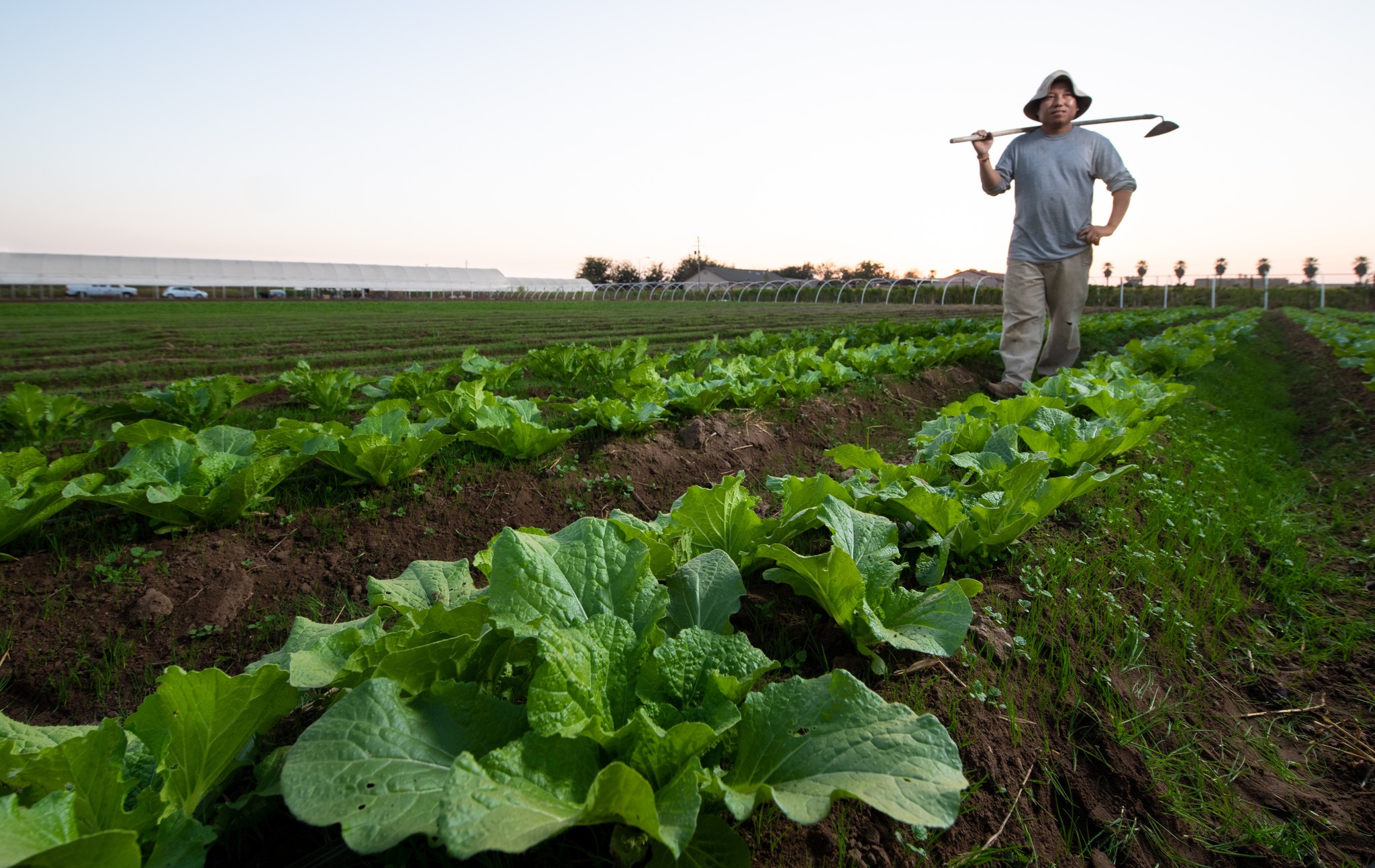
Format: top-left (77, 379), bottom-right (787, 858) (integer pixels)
top-left (985, 380), bottom-right (1025, 399)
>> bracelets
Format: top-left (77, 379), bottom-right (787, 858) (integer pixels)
top-left (977, 155), bottom-right (989, 164)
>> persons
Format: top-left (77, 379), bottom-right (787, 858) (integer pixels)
top-left (971, 70), bottom-right (1137, 398)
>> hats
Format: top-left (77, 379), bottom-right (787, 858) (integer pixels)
top-left (1023, 70), bottom-right (1092, 123)
